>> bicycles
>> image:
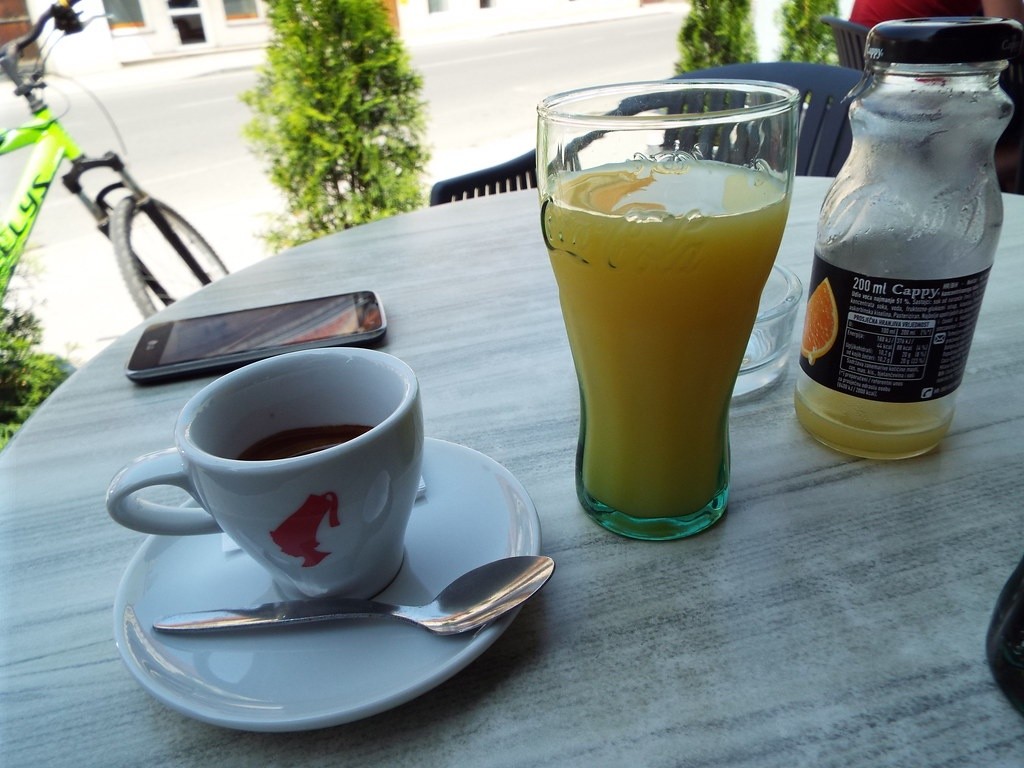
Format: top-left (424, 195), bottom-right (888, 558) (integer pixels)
top-left (1, 0), bottom-right (235, 320)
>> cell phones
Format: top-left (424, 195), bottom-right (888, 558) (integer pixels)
top-left (124, 291), bottom-right (387, 386)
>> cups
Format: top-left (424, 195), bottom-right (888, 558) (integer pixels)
top-left (536, 78), bottom-right (800, 540)
top-left (733, 261), bottom-right (804, 397)
top-left (106, 347), bottom-right (423, 602)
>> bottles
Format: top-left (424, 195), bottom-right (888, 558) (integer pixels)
top-left (795, 17), bottom-right (1023, 459)
top-left (985, 557), bottom-right (1024, 715)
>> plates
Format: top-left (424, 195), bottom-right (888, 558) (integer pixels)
top-left (112, 438), bottom-right (543, 732)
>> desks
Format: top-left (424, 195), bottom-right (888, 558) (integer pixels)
top-left (0, 181), bottom-right (1024, 768)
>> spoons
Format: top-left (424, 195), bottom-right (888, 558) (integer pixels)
top-left (152, 555), bottom-right (556, 635)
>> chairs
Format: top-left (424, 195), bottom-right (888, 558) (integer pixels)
top-left (430, 16), bottom-right (1023, 207)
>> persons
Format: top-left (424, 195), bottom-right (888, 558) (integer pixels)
top-left (850, 0), bottom-right (1024, 194)
